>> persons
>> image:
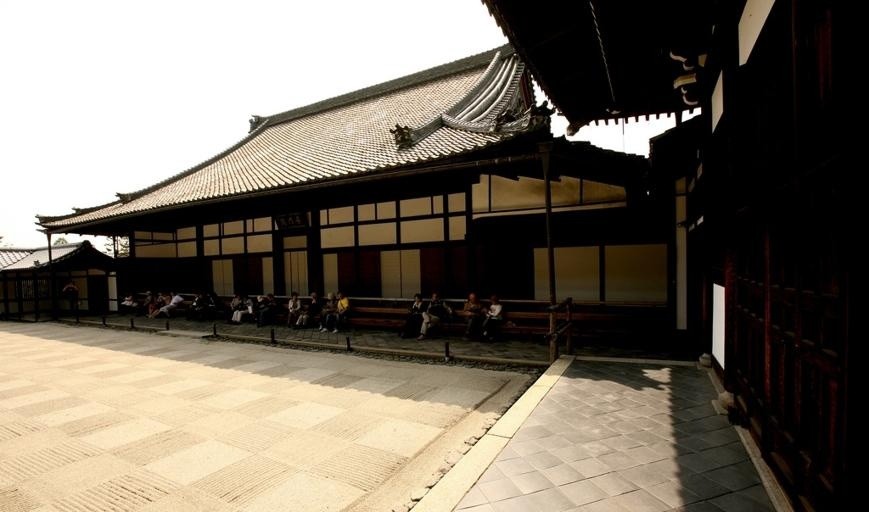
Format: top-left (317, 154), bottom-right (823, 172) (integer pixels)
top-left (119, 289), bottom-right (354, 335)
top-left (416, 291), bottom-right (451, 340)
top-left (407, 293), bottom-right (426, 315)
top-left (479, 294), bottom-right (508, 342)
top-left (450, 293), bottom-right (482, 340)
